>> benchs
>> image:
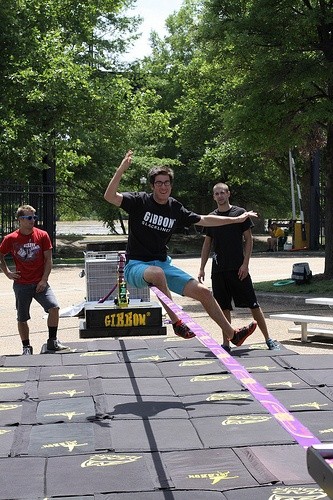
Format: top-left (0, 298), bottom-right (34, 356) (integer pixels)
top-left (79, 240), bottom-right (129, 251)
top-left (269, 298), bottom-right (333, 342)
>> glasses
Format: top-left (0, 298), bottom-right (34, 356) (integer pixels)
top-left (154, 180), bottom-right (171, 186)
top-left (19, 215), bottom-right (39, 220)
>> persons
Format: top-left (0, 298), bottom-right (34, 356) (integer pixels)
top-left (104, 149), bottom-right (258, 347)
top-left (0, 204), bottom-right (70, 355)
top-left (198, 183), bottom-right (280, 353)
top-left (267, 222), bottom-right (285, 253)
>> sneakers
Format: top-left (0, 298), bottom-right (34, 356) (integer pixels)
top-left (172, 320), bottom-right (196, 338)
top-left (46, 337), bottom-right (71, 353)
top-left (22, 345), bottom-right (33, 355)
top-left (230, 321), bottom-right (257, 346)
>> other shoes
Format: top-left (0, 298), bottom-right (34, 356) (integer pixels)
top-left (221, 345), bottom-right (231, 353)
top-left (266, 338), bottom-right (277, 350)
